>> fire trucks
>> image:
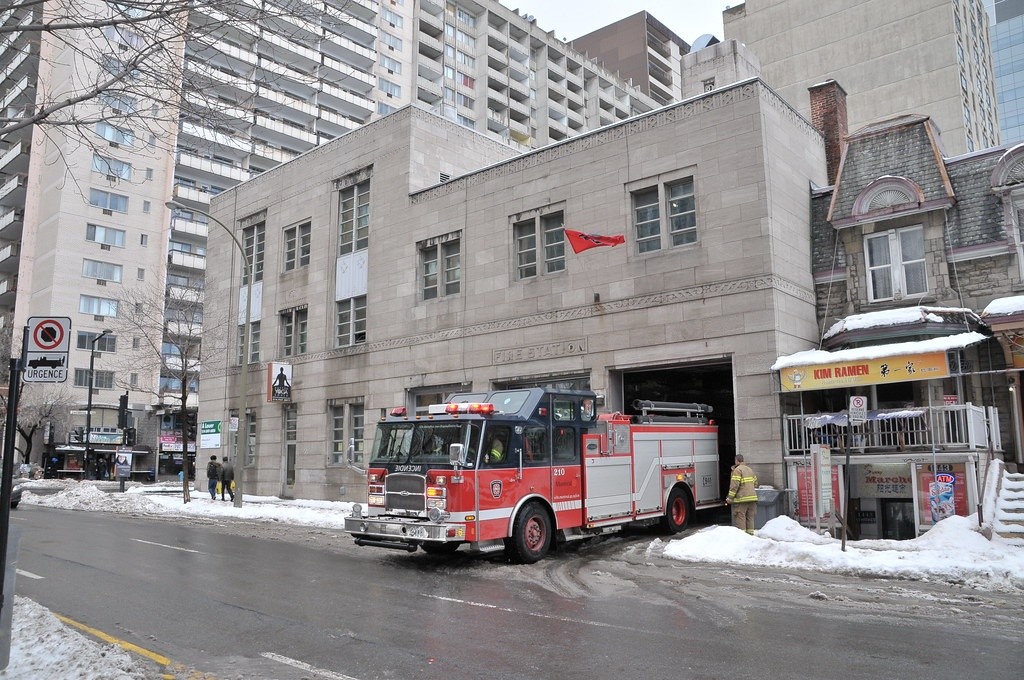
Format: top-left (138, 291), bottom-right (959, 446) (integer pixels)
top-left (344, 384), bottom-right (726, 566)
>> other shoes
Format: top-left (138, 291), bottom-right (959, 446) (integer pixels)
top-left (230, 495), bottom-right (234, 501)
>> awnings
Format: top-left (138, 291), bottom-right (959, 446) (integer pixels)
top-left (774, 331), bottom-right (992, 393)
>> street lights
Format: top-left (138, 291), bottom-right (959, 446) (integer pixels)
top-left (84, 329), bottom-right (112, 482)
top-left (165, 199), bottom-right (253, 509)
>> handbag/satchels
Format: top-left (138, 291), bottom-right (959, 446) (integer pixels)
top-left (208, 463), bottom-right (218, 478)
top-left (230, 480), bottom-right (236, 491)
top-left (216, 481), bottom-right (222, 494)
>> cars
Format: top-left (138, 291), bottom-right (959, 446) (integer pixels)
top-left (0, 463), bottom-right (23, 509)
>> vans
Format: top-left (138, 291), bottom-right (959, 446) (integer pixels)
top-left (159, 451), bottom-right (184, 475)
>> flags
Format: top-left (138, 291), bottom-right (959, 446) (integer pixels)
top-left (565, 228), bottom-right (625, 254)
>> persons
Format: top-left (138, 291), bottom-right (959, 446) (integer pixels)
top-left (220, 456), bottom-right (234, 501)
top-left (484, 431), bottom-right (503, 463)
top-left (207, 454), bottom-right (221, 500)
top-left (726, 454), bottom-right (759, 536)
top-left (116, 455), bottom-right (128, 465)
